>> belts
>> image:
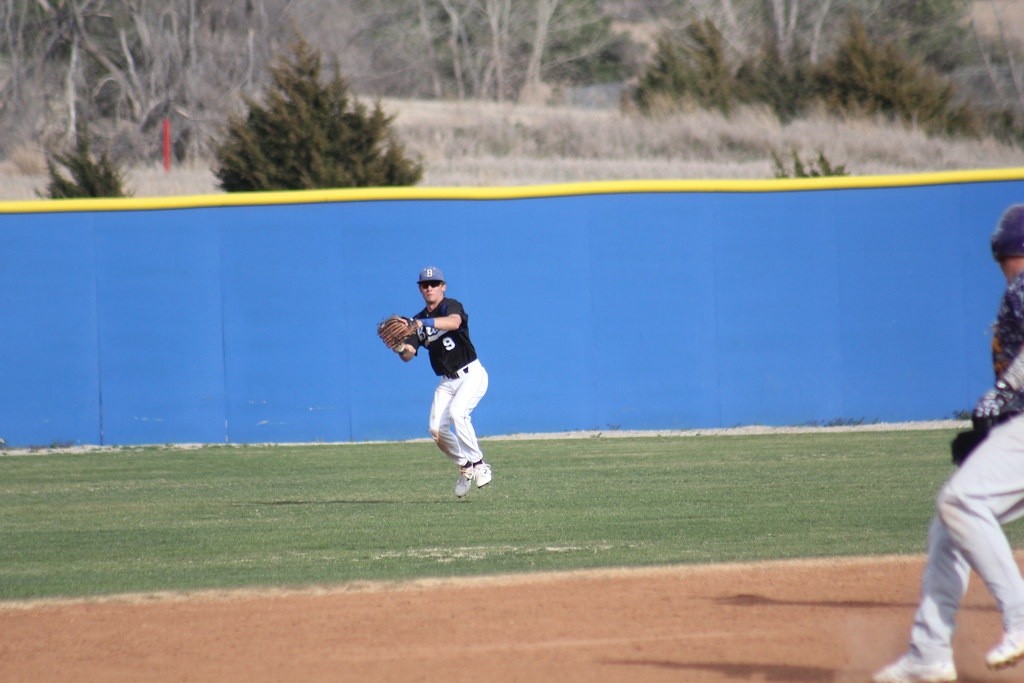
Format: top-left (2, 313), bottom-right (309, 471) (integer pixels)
top-left (443, 367), bottom-right (469, 380)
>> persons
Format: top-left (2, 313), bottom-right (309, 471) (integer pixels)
top-left (377, 266), bottom-right (492, 497)
top-left (872, 203), bottom-right (1024, 683)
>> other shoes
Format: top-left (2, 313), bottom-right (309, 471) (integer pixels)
top-left (986, 631), bottom-right (1024, 671)
top-left (871, 656), bottom-right (958, 683)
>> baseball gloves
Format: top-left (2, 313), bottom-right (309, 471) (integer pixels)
top-left (377, 316), bottom-right (420, 350)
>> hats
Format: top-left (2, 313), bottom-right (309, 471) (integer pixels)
top-left (991, 205), bottom-right (1024, 260)
top-left (417, 267), bottom-right (444, 281)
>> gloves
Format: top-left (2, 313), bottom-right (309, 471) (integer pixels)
top-left (976, 382), bottom-right (1016, 419)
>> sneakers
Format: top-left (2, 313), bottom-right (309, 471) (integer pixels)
top-left (472, 461), bottom-right (492, 488)
top-left (454, 464), bottom-right (473, 497)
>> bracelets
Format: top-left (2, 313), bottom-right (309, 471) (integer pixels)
top-left (419, 318), bottom-right (435, 328)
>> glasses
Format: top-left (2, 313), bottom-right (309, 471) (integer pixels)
top-left (421, 280), bottom-right (442, 288)
